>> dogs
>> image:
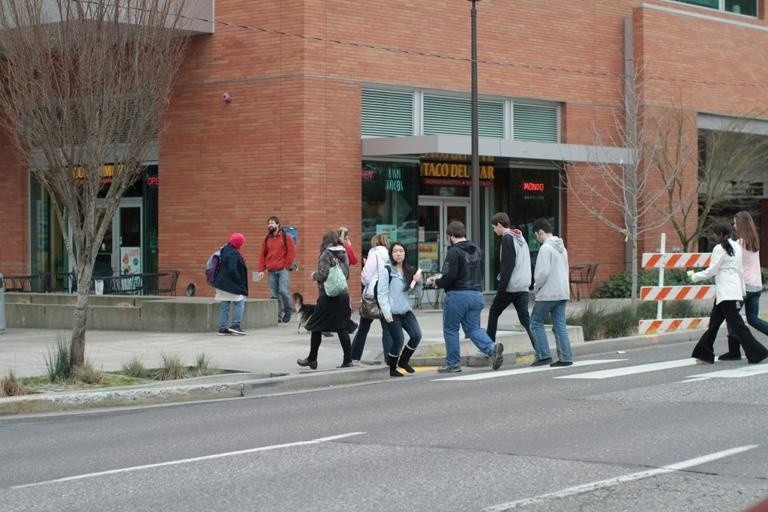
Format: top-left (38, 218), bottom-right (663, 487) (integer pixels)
top-left (293, 292), bottom-right (333, 338)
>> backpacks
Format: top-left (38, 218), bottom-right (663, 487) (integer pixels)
top-left (205, 244), bottom-right (229, 287)
top-left (265, 225), bottom-right (299, 272)
top-left (373, 263), bottom-right (394, 310)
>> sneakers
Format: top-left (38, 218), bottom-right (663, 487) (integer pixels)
top-left (228, 325), bottom-right (249, 336)
top-left (436, 364), bottom-right (463, 374)
top-left (218, 326), bottom-right (231, 336)
top-left (490, 341), bottom-right (505, 371)
top-left (352, 359), bottom-right (361, 367)
top-left (549, 360), bottom-right (574, 367)
top-left (282, 313), bottom-right (293, 323)
top-left (530, 356), bottom-right (553, 367)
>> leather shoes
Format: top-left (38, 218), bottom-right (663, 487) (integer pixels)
top-left (297, 358), bottom-right (318, 369)
top-left (336, 362), bottom-right (354, 368)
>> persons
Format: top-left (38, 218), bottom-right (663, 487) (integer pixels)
top-left (431, 220), bottom-right (504, 373)
top-left (686, 222), bottom-right (768, 364)
top-left (487, 212), bottom-right (535, 349)
top-left (718, 211), bottom-right (768, 360)
top-left (351, 234), bottom-right (393, 365)
top-left (258, 216), bottom-right (297, 323)
top-left (337, 226), bottom-right (358, 266)
top-left (529, 217), bottom-right (573, 367)
top-left (210, 232), bottom-right (248, 336)
top-left (376, 242), bottom-right (424, 377)
top-left (297, 229), bottom-right (355, 370)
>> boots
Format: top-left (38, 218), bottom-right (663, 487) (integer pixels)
top-left (398, 344), bottom-right (416, 374)
top-left (388, 352), bottom-right (404, 377)
top-left (718, 335), bottom-right (742, 361)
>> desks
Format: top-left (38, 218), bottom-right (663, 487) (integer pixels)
top-left (568, 265), bottom-right (584, 270)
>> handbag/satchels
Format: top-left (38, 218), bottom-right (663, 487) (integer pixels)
top-left (323, 259), bottom-right (349, 298)
top-left (358, 292), bottom-right (382, 319)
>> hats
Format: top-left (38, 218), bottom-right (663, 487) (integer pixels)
top-left (228, 233), bottom-right (246, 249)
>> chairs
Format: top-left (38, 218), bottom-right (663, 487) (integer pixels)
top-left (569, 261), bottom-right (599, 301)
top-left (415, 271), bottom-right (444, 309)
top-left (0, 271), bottom-right (181, 296)
